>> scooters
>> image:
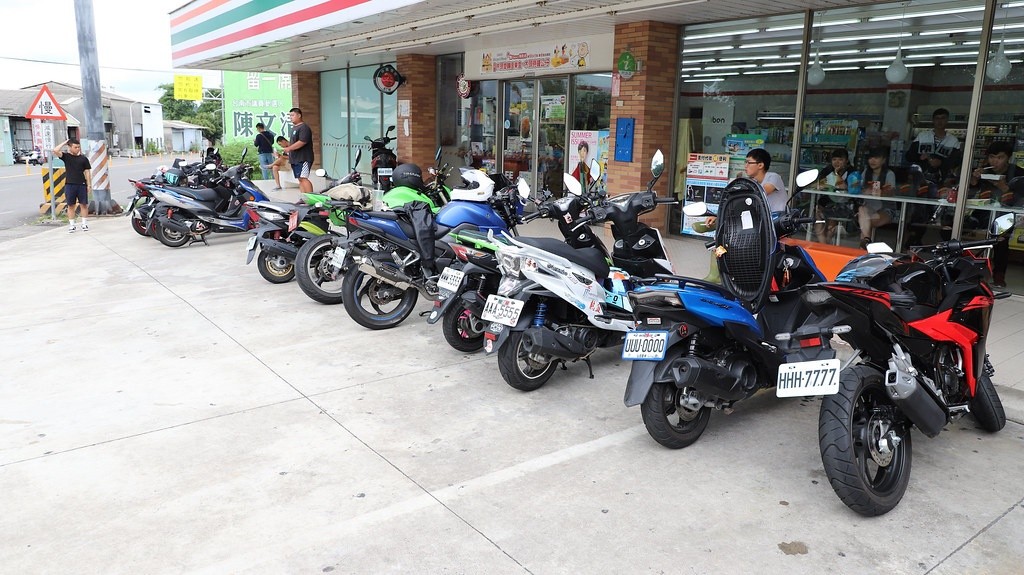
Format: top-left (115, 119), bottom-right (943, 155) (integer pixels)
top-left (31, 151), bottom-right (44, 166)
top-left (362, 123), bottom-right (402, 194)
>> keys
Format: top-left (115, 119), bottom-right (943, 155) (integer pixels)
top-left (783, 258), bottom-right (789, 270)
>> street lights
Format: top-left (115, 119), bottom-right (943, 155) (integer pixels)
top-left (129, 100), bottom-right (143, 158)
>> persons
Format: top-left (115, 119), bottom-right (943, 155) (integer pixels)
top-left (904, 108), bottom-right (961, 183)
top-left (53, 138), bottom-right (92, 234)
top-left (281, 108), bottom-right (315, 203)
top-left (941, 141), bottom-right (1024, 287)
top-left (809, 148), bottom-right (859, 246)
top-left (252, 123), bottom-right (275, 181)
top-left (899, 164), bottom-right (938, 253)
top-left (692, 148), bottom-right (790, 286)
top-left (571, 141), bottom-right (592, 185)
top-left (857, 149), bottom-right (896, 250)
top-left (262, 136), bottom-right (290, 190)
top-left (35, 146), bottom-right (41, 158)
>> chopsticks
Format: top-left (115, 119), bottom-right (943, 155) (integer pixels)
top-left (974, 166), bottom-right (995, 172)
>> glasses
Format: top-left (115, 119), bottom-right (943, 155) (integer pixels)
top-left (744, 161), bottom-right (759, 165)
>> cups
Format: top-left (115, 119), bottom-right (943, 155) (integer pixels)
top-left (369, 190), bottom-right (385, 211)
top-left (873, 181), bottom-right (880, 191)
top-left (522, 124), bottom-right (530, 138)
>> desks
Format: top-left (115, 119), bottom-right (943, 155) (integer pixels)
top-left (800, 188), bottom-right (1024, 242)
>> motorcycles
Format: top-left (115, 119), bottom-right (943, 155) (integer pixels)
top-left (772, 210), bottom-right (1016, 516)
top-left (234, 146), bottom-right (364, 284)
top-left (124, 147), bottom-right (272, 248)
top-left (292, 147), bottom-right (455, 306)
top-left (621, 169), bottom-right (830, 451)
top-left (487, 150), bottom-right (677, 393)
top-left (425, 158), bottom-right (606, 353)
top-left (326, 151), bottom-right (528, 330)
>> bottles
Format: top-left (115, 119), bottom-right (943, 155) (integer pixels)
top-left (847, 172), bottom-right (861, 194)
top-left (948, 187), bottom-right (957, 203)
top-left (992, 124), bottom-right (1024, 143)
top-left (802, 121), bottom-right (851, 143)
top-left (770, 122), bottom-right (794, 145)
top-left (976, 135), bottom-right (992, 144)
top-left (773, 148), bottom-right (832, 164)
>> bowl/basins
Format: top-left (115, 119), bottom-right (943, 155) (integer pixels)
top-left (981, 174), bottom-right (1006, 180)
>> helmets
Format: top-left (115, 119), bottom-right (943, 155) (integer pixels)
top-left (605, 267), bottom-right (632, 313)
top-left (835, 254), bottom-right (916, 309)
top-left (164, 169), bottom-right (187, 186)
top-left (450, 169), bottom-right (495, 201)
top-left (392, 164), bottom-right (423, 189)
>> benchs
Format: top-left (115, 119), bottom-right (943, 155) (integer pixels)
top-left (278, 169), bottom-right (327, 192)
top-left (824, 216), bottom-right (952, 246)
top-left (770, 238), bottom-right (868, 283)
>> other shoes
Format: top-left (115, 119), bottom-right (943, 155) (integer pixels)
top-left (80, 225), bottom-right (89, 231)
top-left (68, 227), bottom-right (75, 232)
top-left (260, 164), bottom-right (272, 169)
top-left (860, 238), bottom-right (871, 249)
top-left (272, 187), bottom-right (282, 191)
top-left (992, 280), bottom-right (1007, 289)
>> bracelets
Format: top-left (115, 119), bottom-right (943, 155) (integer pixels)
top-left (87, 185), bottom-right (91, 187)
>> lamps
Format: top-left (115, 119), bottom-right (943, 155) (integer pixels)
top-left (983, 4), bottom-right (1012, 81)
top-left (805, 11), bottom-right (826, 85)
top-left (885, 3), bottom-right (909, 83)
